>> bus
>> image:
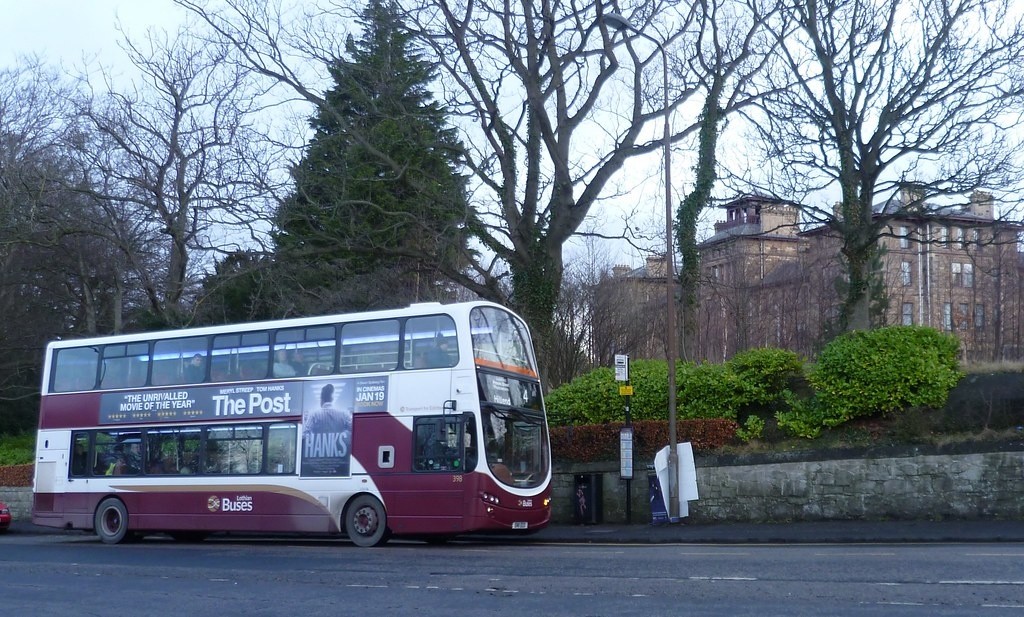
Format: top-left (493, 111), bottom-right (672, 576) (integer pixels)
top-left (31, 301), bottom-right (553, 548)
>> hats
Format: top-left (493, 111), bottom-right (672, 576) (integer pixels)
top-left (436, 332), bottom-right (448, 345)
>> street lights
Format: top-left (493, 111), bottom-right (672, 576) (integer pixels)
top-left (600, 12), bottom-right (681, 523)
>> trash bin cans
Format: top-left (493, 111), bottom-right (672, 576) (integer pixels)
top-left (574, 473), bottom-right (603, 524)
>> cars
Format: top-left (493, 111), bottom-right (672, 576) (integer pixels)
top-left (0, 503), bottom-right (10, 531)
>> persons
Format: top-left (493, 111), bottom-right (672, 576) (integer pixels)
top-left (75, 444), bottom-right (291, 475)
top-left (424, 422), bottom-right (472, 470)
top-left (303, 384), bottom-right (353, 446)
top-left (428, 334), bottom-right (453, 367)
top-left (274, 349), bottom-right (312, 377)
top-left (185, 354), bottom-right (202, 382)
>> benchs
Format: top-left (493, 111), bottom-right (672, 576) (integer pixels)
top-left (69, 362), bottom-right (333, 391)
